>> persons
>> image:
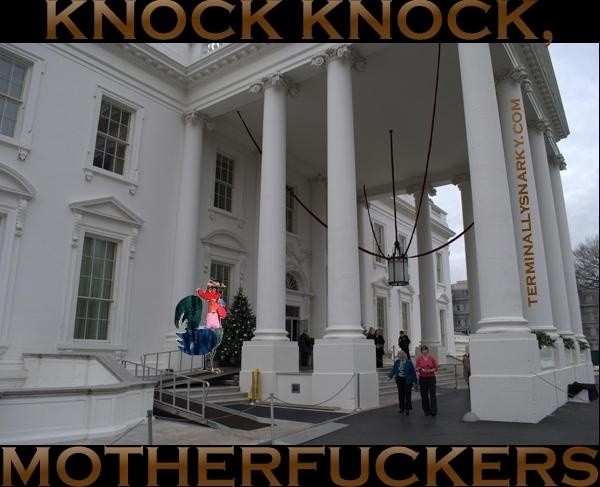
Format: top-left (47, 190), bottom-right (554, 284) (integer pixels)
top-left (397, 331), bottom-right (411, 360)
top-left (384, 350), bottom-right (419, 415)
top-left (414, 345), bottom-right (438, 416)
top-left (373, 330), bottom-right (385, 368)
top-left (297, 329), bottom-right (312, 367)
top-left (462, 353), bottom-right (470, 390)
top-left (366, 327), bottom-right (375, 340)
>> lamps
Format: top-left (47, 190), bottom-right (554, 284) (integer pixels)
top-left (236, 43), bottom-right (474, 286)
